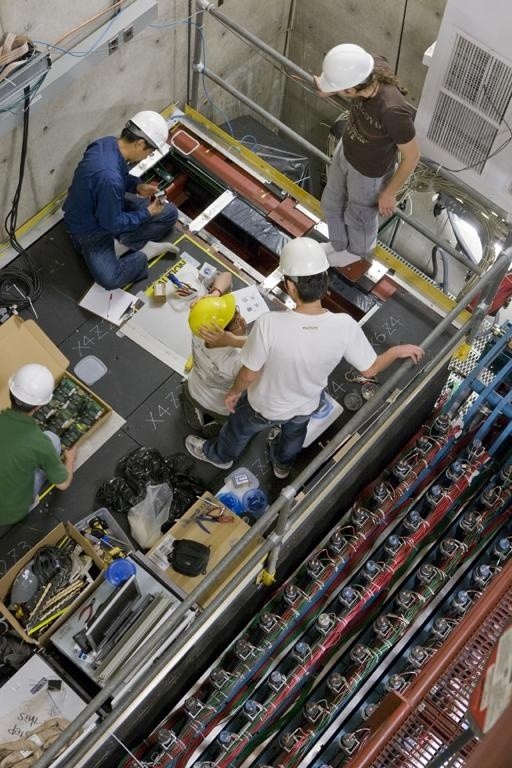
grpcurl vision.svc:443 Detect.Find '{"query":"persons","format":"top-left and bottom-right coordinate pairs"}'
top-left (0, 362), bottom-right (77, 525)
top-left (185, 237), bottom-right (421, 479)
top-left (311, 44), bottom-right (420, 269)
top-left (61, 110), bottom-right (180, 290)
top-left (177, 271), bottom-right (250, 440)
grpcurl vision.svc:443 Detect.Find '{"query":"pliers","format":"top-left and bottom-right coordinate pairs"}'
top-left (194, 517), bottom-right (217, 533)
top-left (176, 290), bottom-right (190, 297)
top-left (78, 597), bottom-right (95, 624)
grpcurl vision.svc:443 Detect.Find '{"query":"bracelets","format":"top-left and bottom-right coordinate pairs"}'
top-left (207, 286), bottom-right (222, 295)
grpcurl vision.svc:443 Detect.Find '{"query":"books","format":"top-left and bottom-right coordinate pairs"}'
top-left (72, 573), bottom-right (200, 696)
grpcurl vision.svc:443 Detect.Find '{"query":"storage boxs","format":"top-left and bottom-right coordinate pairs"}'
top-left (50, 557), bottom-right (192, 698)
top-left (0, 519), bottom-right (109, 646)
top-left (1, 314), bottom-right (112, 460)
top-left (74, 508), bottom-right (135, 566)
top-left (0, 653), bottom-right (99, 767)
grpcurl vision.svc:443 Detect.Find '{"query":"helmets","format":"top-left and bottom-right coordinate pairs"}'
top-left (130, 110), bottom-right (167, 155)
top-left (319, 42), bottom-right (375, 92)
top-left (8, 364), bottom-right (55, 406)
top-left (188, 294), bottom-right (236, 339)
top-left (276, 236), bottom-right (330, 277)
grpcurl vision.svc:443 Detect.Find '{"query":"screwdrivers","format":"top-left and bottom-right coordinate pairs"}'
top-left (175, 515), bottom-right (234, 523)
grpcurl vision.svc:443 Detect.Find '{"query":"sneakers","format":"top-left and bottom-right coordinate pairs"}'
top-left (179, 393), bottom-right (234, 470)
top-left (267, 428), bottom-right (291, 478)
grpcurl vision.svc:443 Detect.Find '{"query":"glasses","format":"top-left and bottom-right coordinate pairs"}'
top-left (228, 305), bottom-right (240, 332)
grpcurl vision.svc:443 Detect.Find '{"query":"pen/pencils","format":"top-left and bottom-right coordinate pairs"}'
top-left (106, 293), bottom-right (112, 319)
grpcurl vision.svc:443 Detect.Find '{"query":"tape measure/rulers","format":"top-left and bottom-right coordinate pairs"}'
top-left (166, 539), bottom-right (211, 577)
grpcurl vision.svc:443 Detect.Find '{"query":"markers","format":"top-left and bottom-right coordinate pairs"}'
top-left (80, 530), bottom-right (101, 545)
